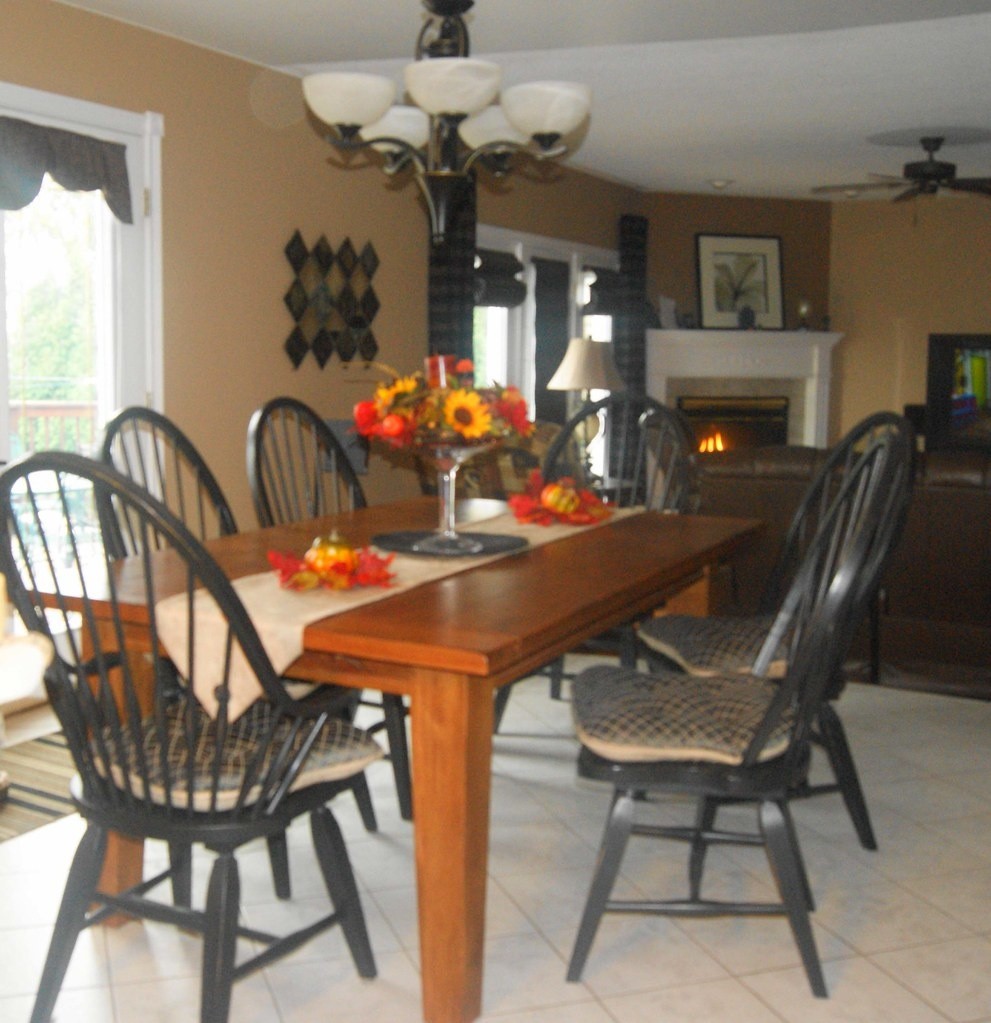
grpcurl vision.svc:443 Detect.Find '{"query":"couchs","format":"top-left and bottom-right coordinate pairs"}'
top-left (665, 445), bottom-right (991, 683)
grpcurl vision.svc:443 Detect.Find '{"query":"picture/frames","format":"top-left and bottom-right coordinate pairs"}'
top-left (695, 232), bottom-right (787, 331)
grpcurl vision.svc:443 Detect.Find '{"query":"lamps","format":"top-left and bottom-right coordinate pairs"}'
top-left (545, 335), bottom-right (626, 483)
top-left (300, 0), bottom-right (590, 250)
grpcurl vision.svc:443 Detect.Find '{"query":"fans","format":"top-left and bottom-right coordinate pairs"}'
top-left (807, 136), bottom-right (991, 205)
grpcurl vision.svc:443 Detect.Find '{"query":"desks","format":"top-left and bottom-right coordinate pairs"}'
top-left (10, 496), bottom-right (763, 1023)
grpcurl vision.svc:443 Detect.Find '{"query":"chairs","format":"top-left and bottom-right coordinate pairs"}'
top-left (246, 399), bottom-right (417, 824)
top-left (490, 394), bottom-right (703, 737)
top-left (0, 449), bottom-right (384, 1023)
top-left (93, 407), bottom-right (378, 900)
top-left (619, 406), bottom-right (919, 851)
top-left (565, 432), bottom-right (899, 1002)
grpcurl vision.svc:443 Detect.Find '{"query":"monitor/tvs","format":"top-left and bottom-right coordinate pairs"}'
top-left (924, 332), bottom-right (991, 453)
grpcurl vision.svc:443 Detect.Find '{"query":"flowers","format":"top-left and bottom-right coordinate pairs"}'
top-left (340, 354), bottom-right (539, 452)
top-left (507, 467), bottom-right (621, 528)
top-left (263, 530), bottom-right (400, 595)
